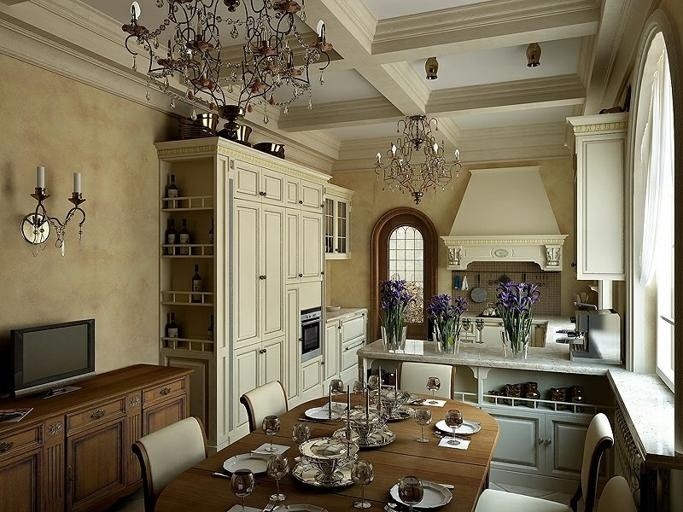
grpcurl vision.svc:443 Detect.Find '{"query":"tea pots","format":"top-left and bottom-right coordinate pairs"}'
top-left (482, 301), bottom-right (497, 317)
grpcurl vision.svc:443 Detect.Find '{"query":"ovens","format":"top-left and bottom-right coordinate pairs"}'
top-left (301, 306), bottom-right (321, 363)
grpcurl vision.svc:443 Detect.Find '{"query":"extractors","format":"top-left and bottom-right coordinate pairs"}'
top-left (435, 165), bottom-right (567, 274)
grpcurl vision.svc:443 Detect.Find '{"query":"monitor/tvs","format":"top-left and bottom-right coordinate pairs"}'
top-left (10, 319), bottom-right (95, 399)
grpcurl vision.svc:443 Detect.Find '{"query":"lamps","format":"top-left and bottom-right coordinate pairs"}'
top-left (525, 42), bottom-right (541, 68)
top-left (373, 116), bottom-right (462, 204)
top-left (423, 57), bottom-right (439, 80)
top-left (120, 0), bottom-right (332, 132)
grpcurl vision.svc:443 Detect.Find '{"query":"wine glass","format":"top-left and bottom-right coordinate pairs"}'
top-left (444, 409), bottom-right (463, 445)
top-left (329, 378), bottom-right (344, 411)
top-left (397, 475), bottom-right (425, 511)
top-left (461, 317), bottom-right (472, 342)
top-left (413, 407), bottom-right (431, 443)
top-left (475, 318), bottom-right (486, 344)
top-left (352, 459), bottom-right (375, 510)
top-left (262, 416), bottom-right (281, 452)
top-left (425, 376), bottom-right (441, 404)
top-left (265, 455), bottom-right (290, 503)
top-left (229, 468), bottom-right (254, 511)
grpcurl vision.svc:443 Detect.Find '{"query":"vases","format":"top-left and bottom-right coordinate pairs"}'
top-left (498, 323), bottom-right (531, 359)
top-left (379, 323), bottom-right (407, 351)
top-left (431, 329), bottom-right (460, 354)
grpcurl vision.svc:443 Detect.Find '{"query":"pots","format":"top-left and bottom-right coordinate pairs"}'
top-left (470, 273), bottom-right (487, 302)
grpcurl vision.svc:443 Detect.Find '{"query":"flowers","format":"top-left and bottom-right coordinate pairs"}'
top-left (375, 277), bottom-right (416, 346)
top-left (424, 292), bottom-right (469, 351)
top-left (493, 279), bottom-right (543, 356)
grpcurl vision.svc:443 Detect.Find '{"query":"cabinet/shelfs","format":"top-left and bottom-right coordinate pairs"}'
top-left (287, 176), bottom-right (324, 284)
top-left (0, 389), bottom-right (68, 512)
top-left (475, 405), bottom-right (601, 497)
top-left (298, 357), bottom-right (323, 406)
top-left (139, 356), bottom-right (195, 512)
top-left (283, 289), bottom-right (299, 412)
top-left (324, 307), bottom-right (368, 396)
top-left (157, 159), bottom-right (215, 352)
top-left (63, 371), bottom-right (139, 511)
top-left (323, 182), bottom-right (355, 262)
top-left (232, 158), bottom-right (288, 441)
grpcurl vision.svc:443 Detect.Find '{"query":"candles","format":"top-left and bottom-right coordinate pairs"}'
top-left (72, 172), bottom-right (80, 192)
top-left (36, 166), bottom-right (44, 188)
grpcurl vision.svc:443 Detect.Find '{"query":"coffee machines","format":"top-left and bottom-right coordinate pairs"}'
top-left (555, 308), bottom-right (623, 367)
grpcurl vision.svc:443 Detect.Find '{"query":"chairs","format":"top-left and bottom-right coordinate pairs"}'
top-left (131, 414), bottom-right (207, 510)
top-left (239, 379), bottom-right (287, 433)
top-left (597, 474), bottom-right (637, 510)
top-left (475, 413), bottom-right (615, 511)
top-left (399, 361), bottom-right (456, 400)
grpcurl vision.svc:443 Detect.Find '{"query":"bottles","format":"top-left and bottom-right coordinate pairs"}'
top-left (190, 262), bottom-right (202, 302)
top-left (207, 315), bottom-right (213, 350)
top-left (165, 215), bottom-right (191, 256)
top-left (207, 217), bottom-right (214, 254)
top-left (167, 172), bottom-right (179, 208)
top-left (524, 380), bottom-right (541, 408)
top-left (167, 312), bottom-right (178, 350)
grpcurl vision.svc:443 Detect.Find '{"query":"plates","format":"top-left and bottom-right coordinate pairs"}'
top-left (434, 419), bottom-right (482, 435)
top-left (223, 452), bottom-right (277, 475)
top-left (389, 480), bottom-right (454, 508)
top-left (305, 407), bottom-right (339, 420)
top-left (271, 503), bottom-right (328, 512)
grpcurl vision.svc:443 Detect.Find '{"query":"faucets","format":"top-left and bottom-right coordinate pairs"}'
top-left (573, 301), bottom-right (597, 311)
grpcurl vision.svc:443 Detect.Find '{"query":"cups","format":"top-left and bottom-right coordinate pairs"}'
top-left (486, 390), bottom-right (503, 403)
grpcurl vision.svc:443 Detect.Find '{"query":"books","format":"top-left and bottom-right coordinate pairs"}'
top-left (0, 408), bottom-right (34, 423)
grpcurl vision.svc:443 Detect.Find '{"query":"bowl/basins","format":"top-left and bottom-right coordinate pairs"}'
top-left (192, 113), bottom-right (285, 158)
